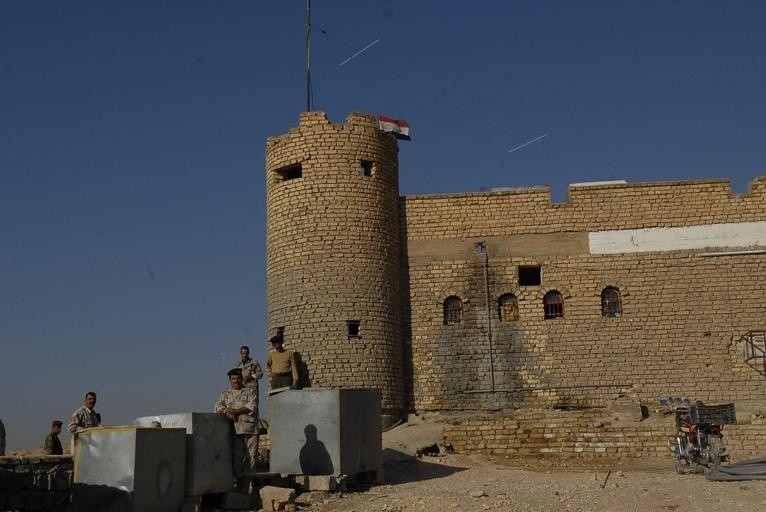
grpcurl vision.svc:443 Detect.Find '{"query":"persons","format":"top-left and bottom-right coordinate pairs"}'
top-left (0, 417), bottom-right (6, 457)
top-left (43, 420), bottom-right (64, 456)
top-left (266, 335), bottom-right (299, 390)
top-left (213, 367), bottom-right (261, 494)
top-left (64, 390), bottom-right (103, 456)
top-left (231, 344), bottom-right (263, 405)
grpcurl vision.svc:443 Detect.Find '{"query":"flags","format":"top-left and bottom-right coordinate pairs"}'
top-left (377, 112), bottom-right (412, 141)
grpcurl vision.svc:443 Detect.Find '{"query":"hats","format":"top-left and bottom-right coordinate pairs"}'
top-left (268, 334), bottom-right (284, 343)
top-left (51, 420), bottom-right (64, 427)
top-left (226, 367), bottom-right (242, 377)
